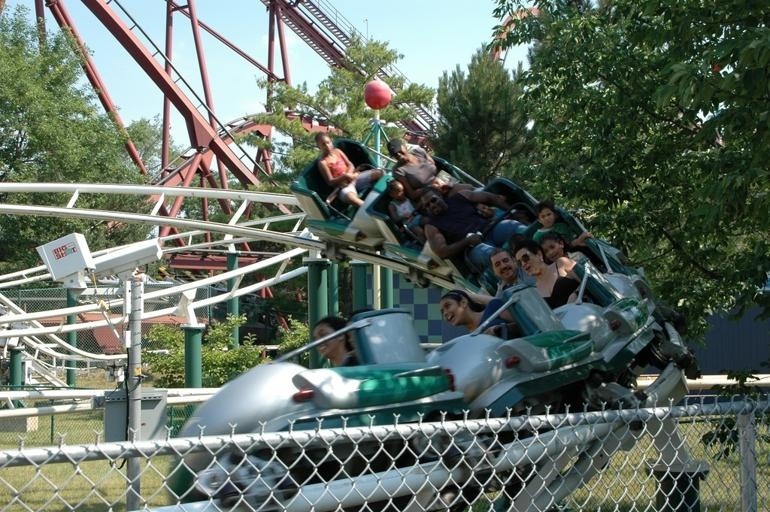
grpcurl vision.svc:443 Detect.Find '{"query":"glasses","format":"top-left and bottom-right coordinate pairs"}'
top-left (424, 195), bottom-right (438, 208)
top-left (519, 252), bottom-right (530, 269)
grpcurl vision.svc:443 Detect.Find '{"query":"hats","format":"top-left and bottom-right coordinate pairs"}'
top-left (387, 139), bottom-right (401, 157)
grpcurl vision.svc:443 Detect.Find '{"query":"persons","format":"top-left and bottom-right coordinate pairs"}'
top-left (315, 132), bottom-right (384, 206)
top-left (115, 366), bottom-right (124, 388)
top-left (313, 317), bottom-right (361, 367)
top-left (386, 139), bottom-right (608, 341)
